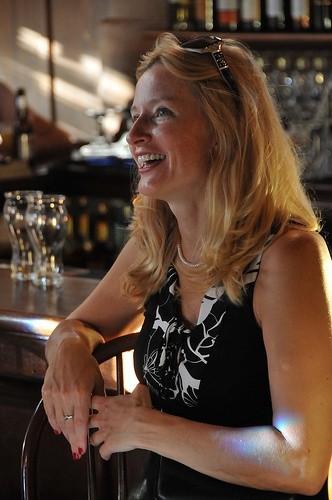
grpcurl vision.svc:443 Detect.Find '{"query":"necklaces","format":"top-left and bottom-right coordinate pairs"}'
top-left (177, 239), bottom-right (214, 267)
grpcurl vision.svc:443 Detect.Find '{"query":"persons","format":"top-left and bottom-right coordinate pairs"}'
top-left (41, 35), bottom-right (332, 500)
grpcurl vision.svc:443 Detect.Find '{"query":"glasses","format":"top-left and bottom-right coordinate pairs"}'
top-left (179, 34), bottom-right (240, 101)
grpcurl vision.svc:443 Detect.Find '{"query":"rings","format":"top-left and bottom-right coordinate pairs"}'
top-left (63, 415), bottom-right (75, 421)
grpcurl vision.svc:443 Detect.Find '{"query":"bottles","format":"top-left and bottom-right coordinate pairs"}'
top-left (254, 49), bottom-right (332, 140)
top-left (78, 195), bottom-right (116, 268)
top-left (12, 89), bottom-right (33, 160)
top-left (167, 0), bottom-right (332, 33)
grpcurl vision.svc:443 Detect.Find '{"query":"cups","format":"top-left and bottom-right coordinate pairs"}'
top-left (25, 194), bottom-right (70, 288)
top-left (3, 190), bottom-right (43, 282)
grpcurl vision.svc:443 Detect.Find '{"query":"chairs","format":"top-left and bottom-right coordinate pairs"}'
top-left (19, 333), bottom-right (156, 500)
